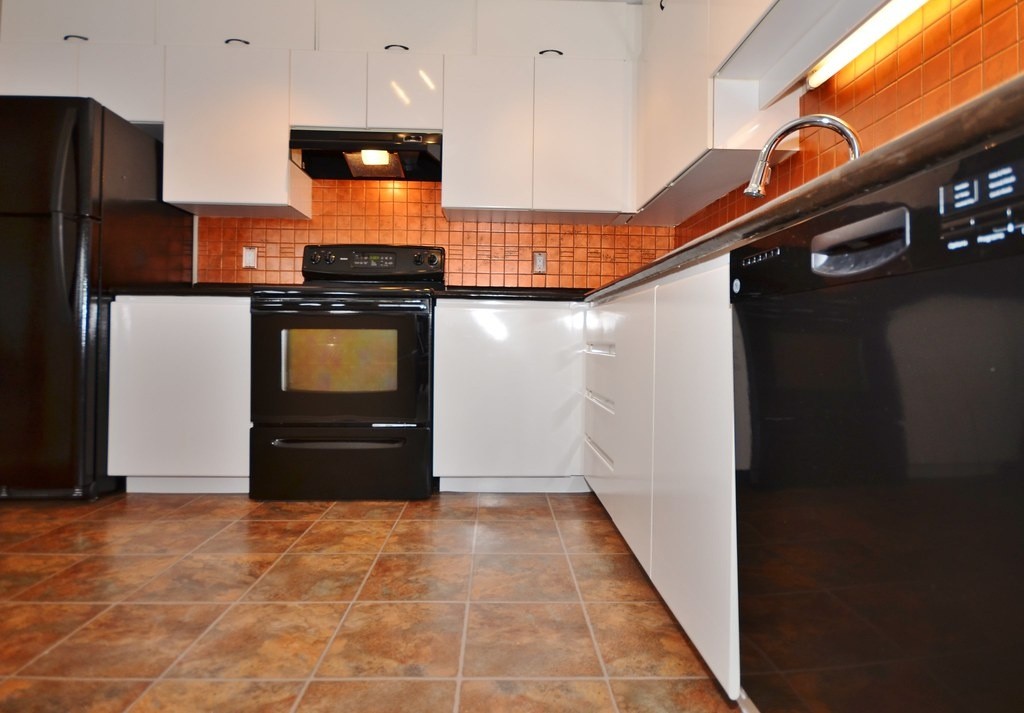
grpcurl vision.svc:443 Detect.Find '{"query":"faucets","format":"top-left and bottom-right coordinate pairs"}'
top-left (743, 113), bottom-right (863, 199)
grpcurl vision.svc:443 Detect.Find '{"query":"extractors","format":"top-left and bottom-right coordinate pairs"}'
top-left (288, 130), bottom-right (442, 182)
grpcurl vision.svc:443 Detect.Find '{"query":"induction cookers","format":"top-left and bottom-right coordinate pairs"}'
top-left (252, 244), bottom-right (444, 298)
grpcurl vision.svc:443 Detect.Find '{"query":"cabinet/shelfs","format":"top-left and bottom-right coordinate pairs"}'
top-left (634, 1), bottom-right (800, 229)
top-left (163, 46), bottom-right (312, 220)
top-left (443, 53), bottom-right (635, 225)
top-left (649, 228), bottom-right (741, 703)
top-left (0, 43), bottom-right (164, 125)
top-left (583, 261), bottom-right (656, 582)
top-left (475, 0), bottom-right (638, 55)
top-left (156, 0), bottom-right (317, 46)
top-left (108, 292), bottom-right (251, 493)
top-left (316, 0), bottom-right (476, 52)
top-left (433, 298), bottom-right (586, 493)
top-left (290, 48), bottom-right (442, 132)
top-left (1, 1), bottom-right (156, 43)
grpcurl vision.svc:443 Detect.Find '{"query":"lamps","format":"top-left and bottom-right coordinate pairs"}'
top-left (805, 0), bottom-right (928, 90)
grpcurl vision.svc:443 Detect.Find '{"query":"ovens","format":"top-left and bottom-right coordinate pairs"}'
top-left (248, 283), bottom-right (434, 500)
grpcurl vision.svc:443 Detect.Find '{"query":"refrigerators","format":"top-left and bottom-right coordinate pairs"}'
top-left (0, 95), bottom-right (163, 503)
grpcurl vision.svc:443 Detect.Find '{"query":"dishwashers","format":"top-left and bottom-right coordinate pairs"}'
top-left (729, 131), bottom-right (1024, 713)
